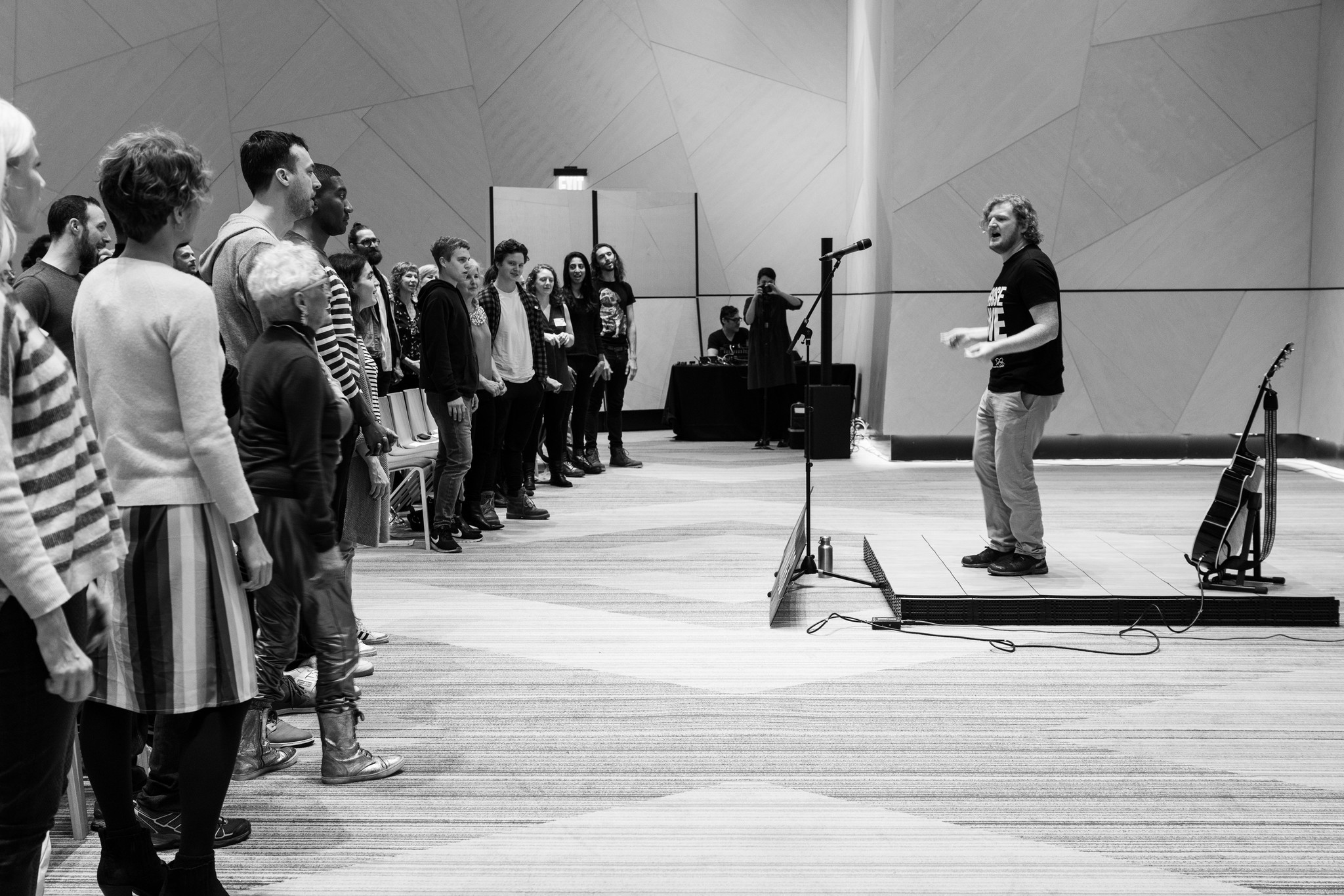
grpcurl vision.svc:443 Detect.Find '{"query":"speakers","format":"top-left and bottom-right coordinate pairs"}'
top-left (805, 384), bottom-right (852, 460)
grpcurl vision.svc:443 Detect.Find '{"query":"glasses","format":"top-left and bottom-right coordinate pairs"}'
top-left (292, 274), bottom-right (329, 294)
top-left (354, 238), bottom-right (380, 248)
top-left (724, 317), bottom-right (742, 323)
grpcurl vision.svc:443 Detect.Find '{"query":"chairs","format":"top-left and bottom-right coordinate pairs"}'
top-left (376, 387), bottom-right (440, 551)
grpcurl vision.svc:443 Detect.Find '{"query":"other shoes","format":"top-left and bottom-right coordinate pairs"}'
top-left (89, 801), bottom-right (108, 831)
top-left (777, 437), bottom-right (793, 447)
top-left (755, 437), bottom-right (770, 446)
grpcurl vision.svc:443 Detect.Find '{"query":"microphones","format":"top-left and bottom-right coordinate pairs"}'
top-left (819, 238), bottom-right (872, 261)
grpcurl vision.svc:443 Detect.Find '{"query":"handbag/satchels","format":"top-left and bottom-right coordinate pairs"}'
top-left (407, 496), bottom-right (434, 531)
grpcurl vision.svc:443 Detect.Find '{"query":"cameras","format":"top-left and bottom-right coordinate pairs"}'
top-left (759, 284), bottom-right (772, 294)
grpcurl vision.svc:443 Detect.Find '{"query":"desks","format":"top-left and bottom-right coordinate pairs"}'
top-left (662, 364), bottom-right (857, 441)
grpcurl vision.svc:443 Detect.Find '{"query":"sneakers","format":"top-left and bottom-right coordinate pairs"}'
top-left (430, 525), bottom-right (462, 552)
top-left (133, 799), bottom-right (251, 849)
top-left (451, 523), bottom-right (483, 541)
top-left (962, 546), bottom-right (1008, 568)
top-left (265, 614), bottom-right (389, 748)
top-left (987, 553), bottom-right (1048, 575)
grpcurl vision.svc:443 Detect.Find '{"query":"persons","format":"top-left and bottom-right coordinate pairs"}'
top-left (525, 264), bottom-right (575, 490)
top-left (559, 251), bottom-right (605, 477)
top-left (584, 243), bottom-right (642, 473)
top-left (707, 305), bottom-right (748, 356)
top-left (0, 92), bottom-right (563, 896)
top-left (743, 267), bottom-right (803, 448)
top-left (940, 194), bottom-right (1064, 576)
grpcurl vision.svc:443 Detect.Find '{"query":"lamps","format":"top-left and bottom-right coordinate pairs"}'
top-left (551, 165), bottom-right (588, 191)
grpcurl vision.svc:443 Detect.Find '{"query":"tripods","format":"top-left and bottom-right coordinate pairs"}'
top-left (766, 260), bottom-right (883, 592)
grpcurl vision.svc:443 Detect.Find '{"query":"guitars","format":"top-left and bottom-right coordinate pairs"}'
top-left (1189, 341), bottom-right (1296, 573)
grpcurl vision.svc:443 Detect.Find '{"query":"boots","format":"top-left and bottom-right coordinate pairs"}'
top-left (480, 491), bottom-right (500, 524)
top-left (586, 447), bottom-right (607, 471)
top-left (523, 462), bottom-right (536, 490)
top-left (161, 850), bottom-right (230, 896)
top-left (461, 501), bottom-right (504, 530)
top-left (493, 484), bottom-right (509, 507)
top-left (231, 708), bottom-right (298, 781)
top-left (571, 448), bottom-right (602, 473)
top-left (609, 446), bottom-right (644, 468)
top-left (97, 828), bottom-right (167, 896)
top-left (455, 499), bottom-right (481, 533)
top-left (548, 455), bottom-right (573, 487)
top-left (506, 486), bottom-right (550, 519)
top-left (562, 450), bottom-right (585, 477)
top-left (317, 708), bottom-right (405, 784)
